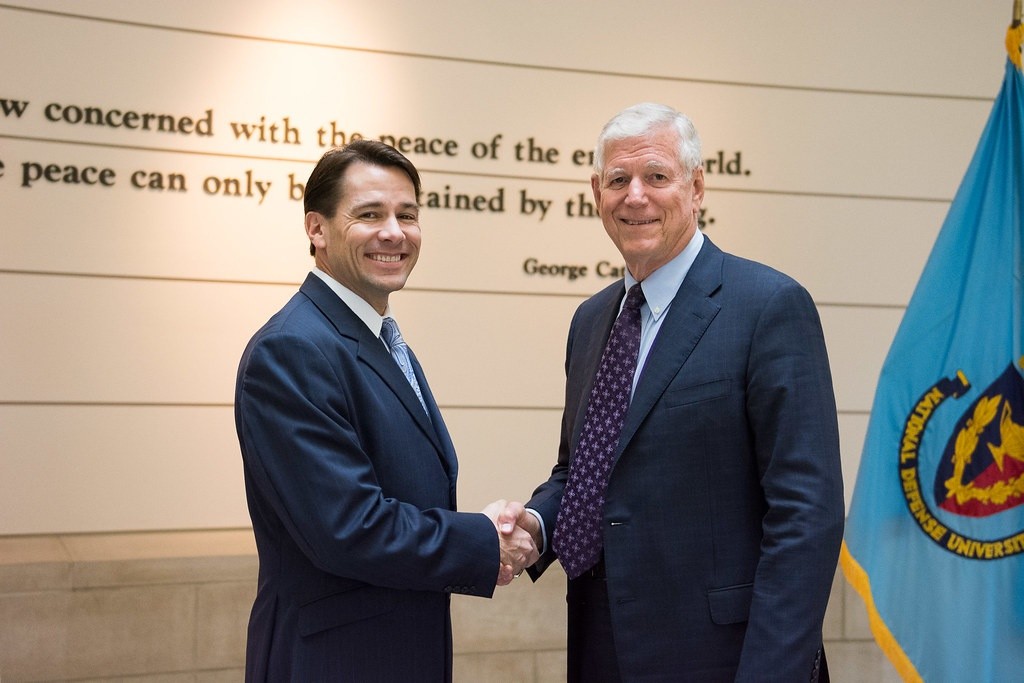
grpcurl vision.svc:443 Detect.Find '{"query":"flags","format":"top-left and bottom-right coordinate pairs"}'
top-left (838, 24), bottom-right (1024, 683)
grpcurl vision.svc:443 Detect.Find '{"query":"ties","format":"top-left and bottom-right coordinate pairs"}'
top-left (380, 317), bottom-right (432, 428)
top-left (553, 283), bottom-right (647, 582)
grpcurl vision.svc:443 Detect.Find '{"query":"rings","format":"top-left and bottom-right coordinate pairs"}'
top-left (514, 569), bottom-right (524, 578)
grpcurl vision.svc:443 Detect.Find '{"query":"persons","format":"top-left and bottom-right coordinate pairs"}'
top-left (234, 141), bottom-right (538, 683)
top-left (497, 102), bottom-right (845, 683)
